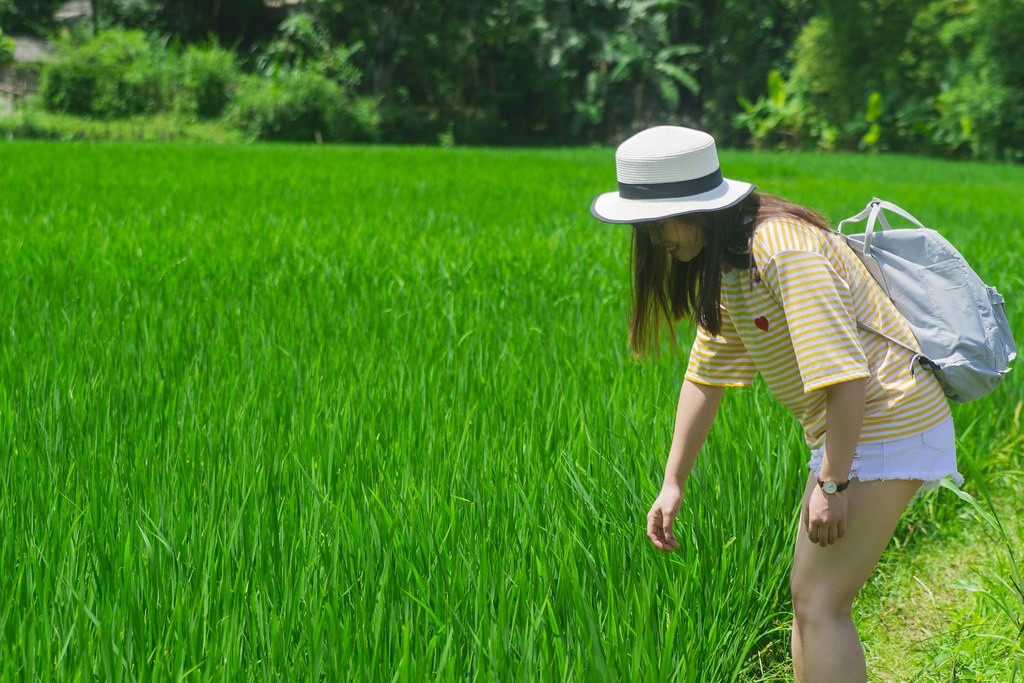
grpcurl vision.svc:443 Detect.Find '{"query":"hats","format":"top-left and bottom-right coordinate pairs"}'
top-left (588, 125), bottom-right (755, 224)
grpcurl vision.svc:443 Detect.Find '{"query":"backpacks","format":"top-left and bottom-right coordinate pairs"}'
top-left (790, 197), bottom-right (1017, 403)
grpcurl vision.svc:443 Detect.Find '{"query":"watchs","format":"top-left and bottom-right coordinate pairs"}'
top-left (816, 477), bottom-right (851, 494)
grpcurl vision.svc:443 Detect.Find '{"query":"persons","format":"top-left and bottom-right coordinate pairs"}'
top-left (591, 126), bottom-right (964, 683)
